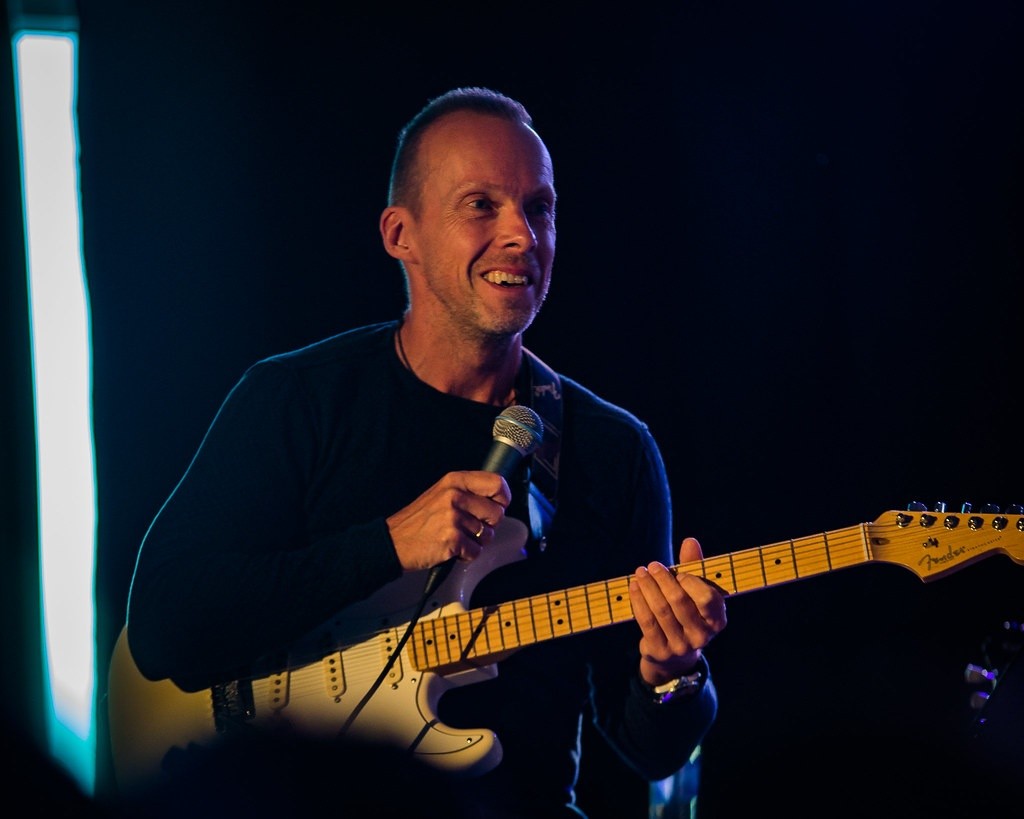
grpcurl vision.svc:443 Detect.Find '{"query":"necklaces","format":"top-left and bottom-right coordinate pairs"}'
top-left (397, 326), bottom-right (517, 407)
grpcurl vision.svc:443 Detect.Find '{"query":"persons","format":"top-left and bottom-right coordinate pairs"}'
top-left (126, 88), bottom-right (728, 819)
top-left (966, 637), bottom-right (1024, 819)
top-left (0, 713), bottom-right (1024, 819)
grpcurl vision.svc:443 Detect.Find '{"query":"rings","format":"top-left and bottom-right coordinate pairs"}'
top-left (476, 521), bottom-right (484, 538)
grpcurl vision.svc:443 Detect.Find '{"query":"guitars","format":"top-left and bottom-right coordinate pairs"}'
top-left (106, 500), bottom-right (1024, 782)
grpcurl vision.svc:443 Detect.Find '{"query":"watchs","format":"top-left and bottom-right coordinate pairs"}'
top-left (641, 656), bottom-right (704, 704)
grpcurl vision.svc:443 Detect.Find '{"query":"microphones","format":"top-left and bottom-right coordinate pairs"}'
top-left (421, 405), bottom-right (542, 596)
